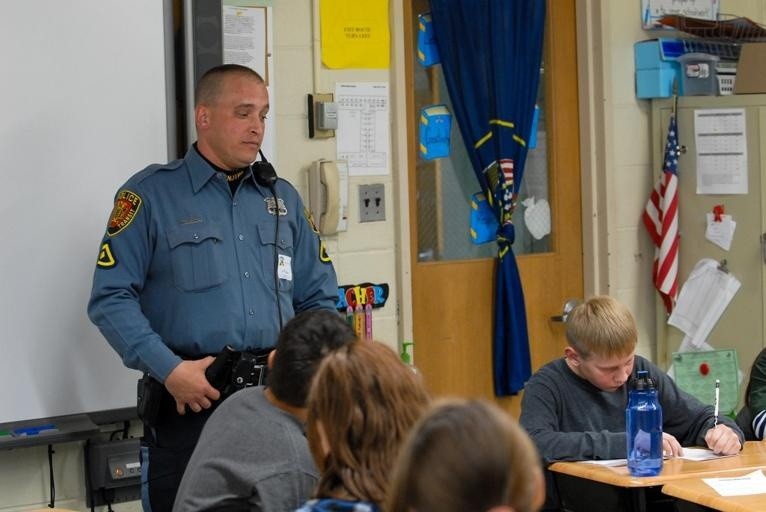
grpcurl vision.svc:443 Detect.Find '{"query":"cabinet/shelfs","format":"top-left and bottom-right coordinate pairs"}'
top-left (649, 95), bottom-right (766, 416)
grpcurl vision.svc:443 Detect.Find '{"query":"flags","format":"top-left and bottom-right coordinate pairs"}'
top-left (635, 100), bottom-right (681, 321)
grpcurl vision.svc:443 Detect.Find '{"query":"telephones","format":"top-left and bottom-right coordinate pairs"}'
top-left (307, 160), bottom-right (350, 236)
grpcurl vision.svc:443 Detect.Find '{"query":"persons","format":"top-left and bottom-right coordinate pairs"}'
top-left (518, 295), bottom-right (747, 468)
top-left (84, 63), bottom-right (342, 511)
top-left (172, 307), bottom-right (359, 512)
top-left (284, 340), bottom-right (434, 512)
top-left (382, 394), bottom-right (547, 512)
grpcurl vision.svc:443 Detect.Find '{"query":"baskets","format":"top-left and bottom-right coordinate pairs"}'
top-left (657, 11), bottom-right (762, 57)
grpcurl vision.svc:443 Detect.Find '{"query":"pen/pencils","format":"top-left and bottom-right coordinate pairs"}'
top-left (714, 380), bottom-right (721, 425)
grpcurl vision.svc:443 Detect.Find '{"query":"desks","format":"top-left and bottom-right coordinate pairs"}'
top-left (548, 439), bottom-right (764, 512)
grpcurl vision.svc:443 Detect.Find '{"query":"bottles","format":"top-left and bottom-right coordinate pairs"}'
top-left (625, 371), bottom-right (663, 477)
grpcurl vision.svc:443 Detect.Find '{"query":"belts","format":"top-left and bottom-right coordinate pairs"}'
top-left (230, 354), bottom-right (268, 390)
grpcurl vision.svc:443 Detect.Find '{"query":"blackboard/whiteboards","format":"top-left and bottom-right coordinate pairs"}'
top-left (2, 5), bottom-right (182, 432)
